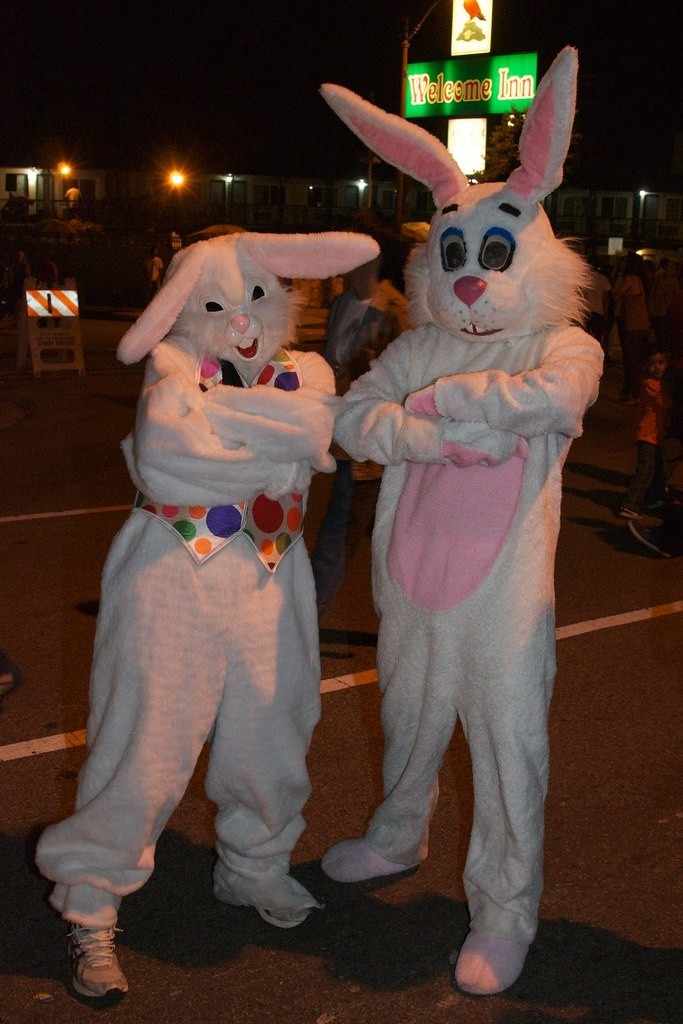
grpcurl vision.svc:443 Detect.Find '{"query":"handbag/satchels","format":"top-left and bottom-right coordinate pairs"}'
top-left (646, 326), bottom-right (656, 345)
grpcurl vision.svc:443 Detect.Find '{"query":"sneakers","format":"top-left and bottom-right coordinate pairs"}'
top-left (214, 885), bottom-right (313, 929)
top-left (628, 519), bottom-right (671, 559)
top-left (68, 923), bottom-right (129, 1002)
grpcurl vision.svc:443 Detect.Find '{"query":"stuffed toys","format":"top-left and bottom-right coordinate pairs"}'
top-left (35, 231), bottom-right (379, 1007)
top-left (321, 46), bottom-right (604, 995)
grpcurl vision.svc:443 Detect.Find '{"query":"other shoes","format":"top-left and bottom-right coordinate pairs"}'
top-left (620, 510), bottom-right (640, 519)
top-left (621, 397), bottom-right (641, 405)
top-left (647, 500), bottom-right (663, 508)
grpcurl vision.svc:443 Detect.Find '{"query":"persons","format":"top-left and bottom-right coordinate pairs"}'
top-left (143, 248), bottom-right (163, 300)
top-left (620, 348), bottom-right (679, 519)
top-left (65, 184), bottom-right (82, 208)
top-left (629, 507), bottom-right (683, 557)
top-left (583, 251), bottom-right (683, 406)
top-left (8, 249), bottom-right (32, 315)
top-left (38, 248), bottom-right (60, 328)
top-left (311, 206), bottom-right (419, 619)
